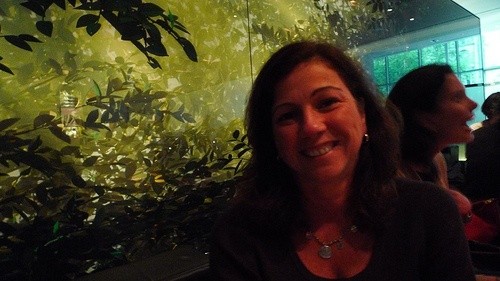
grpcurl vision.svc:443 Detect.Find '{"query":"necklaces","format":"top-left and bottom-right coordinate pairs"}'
top-left (299, 216), bottom-right (359, 259)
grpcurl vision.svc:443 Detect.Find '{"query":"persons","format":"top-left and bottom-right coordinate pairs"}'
top-left (466, 91), bottom-right (500, 197)
top-left (207, 36), bottom-right (477, 281)
top-left (384, 62), bottom-right (479, 224)
